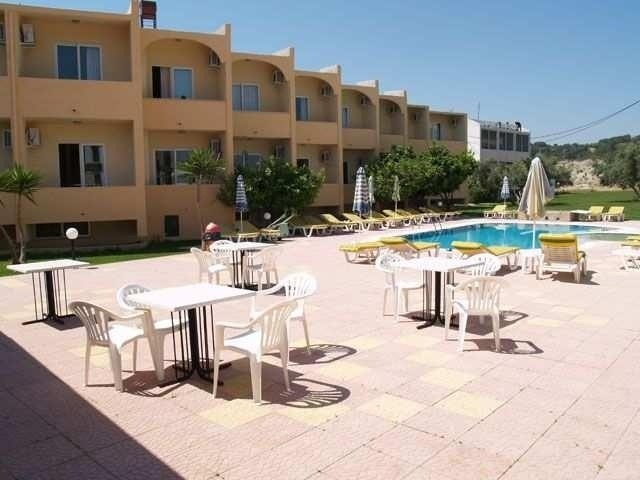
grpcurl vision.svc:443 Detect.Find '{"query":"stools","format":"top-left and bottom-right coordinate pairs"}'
top-left (622, 233), bottom-right (640, 248)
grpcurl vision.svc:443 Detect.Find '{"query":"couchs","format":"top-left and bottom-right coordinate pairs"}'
top-left (290, 216), bottom-right (329, 237)
top-left (320, 213), bottom-right (357, 234)
top-left (587, 206), bottom-right (604, 222)
top-left (217, 223), bottom-right (258, 243)
top-left (483, 205), bottom-right (507, 218)
top-left (381, 237), bottom-right (440, 258)
top-left (451, 239), bottom-right (521, 271)
top-left (361, 206), bottom-right (464, 230)
top-left (342, 213), bottom-right (375, 231)
top-left (233, 221), bottom-right (281, 241)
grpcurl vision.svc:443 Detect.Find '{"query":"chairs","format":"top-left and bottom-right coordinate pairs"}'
top-left (443, 277), bottom-right (505, 353)
top-left (535, 232), bottom-right (588, 284)
top-left (67, 301), bottom-right (166, 392)
top-left (242, 245), bottom-right (282, 292)
top-left (190, 247), bottom-right (236, 289)
top-left (210, 240), bottom-right (240, 285)
top-left (117, 284), bottom-right (195, 381)
top-left (252, 272), bottom-right (317, 357)
top-left (376, 251), bottom-right (425, 317)
top-left (210, 300), bottom-right (299, 404)
top-left (465, 253), bottom-right (501, 283)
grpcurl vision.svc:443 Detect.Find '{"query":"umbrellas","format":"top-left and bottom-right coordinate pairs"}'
top-left (518, 157), bottom-right (554, 248)
top-left (391, 175), bottom-right (401, 216)
top-left (500, 175), bottom-right (510, 211)
top-left (368, 176), bottom-right (376, 217)
top-left (235, 174), bottom-right (250, 232)
top-left (352, 166), bottom-right (371, 221)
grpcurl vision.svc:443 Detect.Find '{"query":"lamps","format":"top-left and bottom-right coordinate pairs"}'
top-left (264, 212), bottom-right (271, 221)
top-left (602, 206), bottom-right (625, 222)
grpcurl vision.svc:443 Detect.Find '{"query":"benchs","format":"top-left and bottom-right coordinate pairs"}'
top-left (339, 242), bottom-right (383, 265)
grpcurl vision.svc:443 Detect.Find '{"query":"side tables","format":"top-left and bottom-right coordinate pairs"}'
top-left (519, 251), bottom-right (540, 276)
top-left (612, 247), bottom-right (640, 270)
